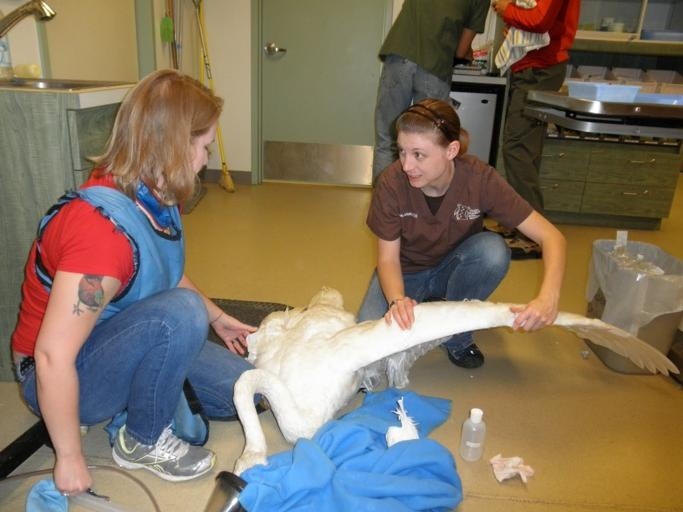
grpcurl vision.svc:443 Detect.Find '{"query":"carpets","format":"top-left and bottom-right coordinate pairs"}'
top-left (183, 299), bottom-right (294, 421)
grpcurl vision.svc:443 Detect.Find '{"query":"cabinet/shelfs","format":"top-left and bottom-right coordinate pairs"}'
top-left (0, 103), bottom-right (120, 383)
top-left (496, 138), bottom-right (683, 231)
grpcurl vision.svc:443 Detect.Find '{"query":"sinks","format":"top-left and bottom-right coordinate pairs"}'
top-left (0, 76), bottom-right (137, 91)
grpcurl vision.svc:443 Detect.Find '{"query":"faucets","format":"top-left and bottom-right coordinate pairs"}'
top-left (0, 0), bottom-right (57, 38)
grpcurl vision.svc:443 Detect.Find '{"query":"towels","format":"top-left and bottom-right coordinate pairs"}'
top-left (238, 388), bottom-right (463, 512)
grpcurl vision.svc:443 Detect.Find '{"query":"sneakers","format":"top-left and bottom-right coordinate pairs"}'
top-left (446, 339), bottom-right (484, 368)
top-left (492, 227), bottom-right (538, 251)
top-left (111, 425), bottom-right (215, 484)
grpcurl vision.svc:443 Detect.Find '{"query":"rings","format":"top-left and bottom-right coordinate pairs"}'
top-left (62, 493), bottom-right (70, 496)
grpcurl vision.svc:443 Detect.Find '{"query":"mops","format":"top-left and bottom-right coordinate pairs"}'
top-left (168, 0), bottom-right (208, 214)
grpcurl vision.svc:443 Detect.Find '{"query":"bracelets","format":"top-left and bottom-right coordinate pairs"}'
top-left (386, 297), bottom-right (404, 308)
top-left (214, 301), bottom-right (224, 325)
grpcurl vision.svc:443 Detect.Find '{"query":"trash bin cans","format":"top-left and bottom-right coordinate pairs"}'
top-left (583, 239), bottom-right (683, 375)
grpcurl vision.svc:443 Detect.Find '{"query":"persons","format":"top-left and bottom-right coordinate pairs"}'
top-left (371, 0), bottom-right (491, 195)
top-left (481, 0), bottom-right (581, 260)
top-left (341, 98), bottom-right (567, 392)
top-left (10, 70), bottom-right (260, 496)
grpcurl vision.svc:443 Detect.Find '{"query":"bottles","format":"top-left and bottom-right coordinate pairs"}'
top-left (459, 408), bottom-right (486, 463)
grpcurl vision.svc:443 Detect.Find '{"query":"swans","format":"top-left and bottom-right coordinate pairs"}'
top-left (231, 283), bottom-right (681, 478)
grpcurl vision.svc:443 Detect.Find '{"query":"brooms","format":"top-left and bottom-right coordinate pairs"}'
top-left (196, 4), bottom-right (237, 193)
